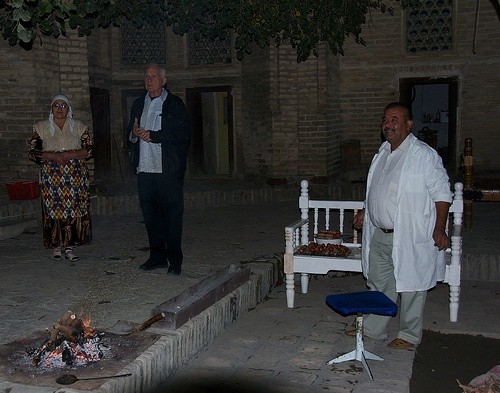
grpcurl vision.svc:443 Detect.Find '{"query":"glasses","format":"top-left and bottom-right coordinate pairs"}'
top-left (54, 104), bottom-right (68, 109)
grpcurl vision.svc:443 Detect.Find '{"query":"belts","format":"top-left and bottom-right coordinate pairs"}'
top-left (380, 228), bottom-right (393, 233)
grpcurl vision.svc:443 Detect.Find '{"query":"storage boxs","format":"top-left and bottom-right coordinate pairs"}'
top-left (6, 179), bottom-right (40, 201)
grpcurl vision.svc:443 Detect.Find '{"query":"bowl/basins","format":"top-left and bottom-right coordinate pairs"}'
top-left (316, 238), bottom-right (343, 245)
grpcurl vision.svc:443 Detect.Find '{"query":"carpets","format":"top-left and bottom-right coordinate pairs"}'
top-left (409, 329), bottom-right (500, 393)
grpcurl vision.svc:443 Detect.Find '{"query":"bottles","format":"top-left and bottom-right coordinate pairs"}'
top-left (422, 111), bottom-right (440, 123)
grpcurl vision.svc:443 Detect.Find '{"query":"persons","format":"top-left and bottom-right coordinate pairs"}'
top-left (30, 95), bottom-right (93, 262)
top-left (346, 102), bottom-right (454, 350)
top-left (125, 63), bottom-right (189, 276)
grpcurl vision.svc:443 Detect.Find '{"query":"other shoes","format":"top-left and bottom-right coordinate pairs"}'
top-left (388, 338), bottom-right (414, 349)
top-left (167, 263), bottom-right (181, 277)
top-left (140, 257), bottom-right (168, 271)
top-left (345, 329), bottom-right (356, 336)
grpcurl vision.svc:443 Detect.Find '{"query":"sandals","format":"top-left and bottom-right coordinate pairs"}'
top-left (65, 251), bottom-right (80, 261)
top-left (52, 249), bottom-right (62, 260)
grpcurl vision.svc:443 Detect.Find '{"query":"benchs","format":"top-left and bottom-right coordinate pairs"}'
top-left (284, 180), bottom-right (463, 322)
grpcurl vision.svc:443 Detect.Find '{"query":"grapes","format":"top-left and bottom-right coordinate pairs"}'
top-left (299, 242), bottom-right (334, 253)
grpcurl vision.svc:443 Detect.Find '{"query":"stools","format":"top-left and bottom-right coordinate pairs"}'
top-left (326, 291), bottom-right (398, 381)
